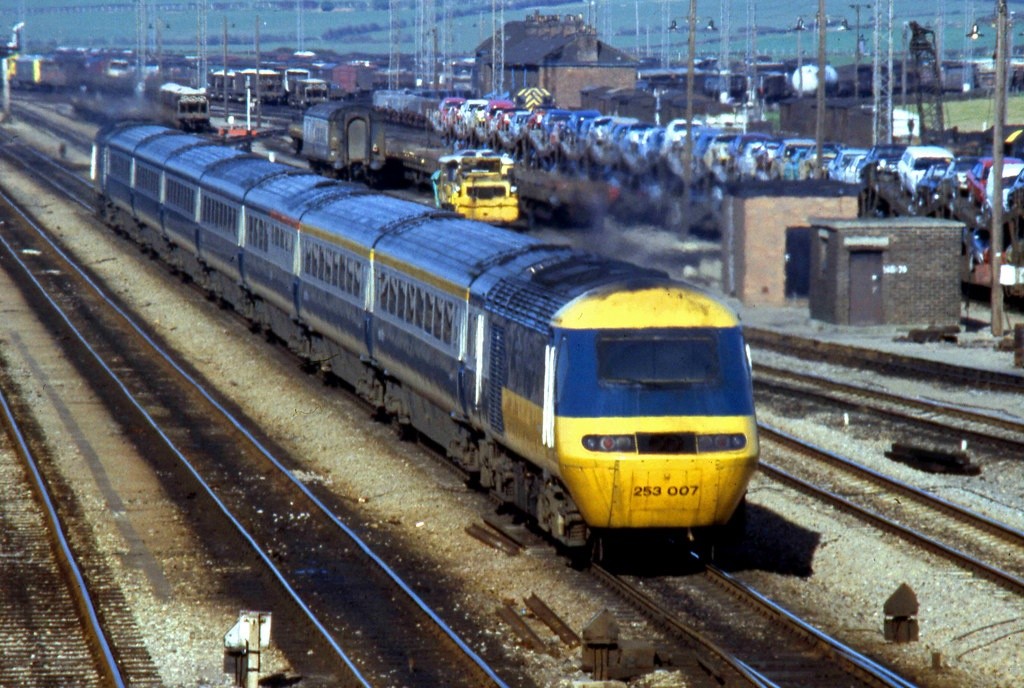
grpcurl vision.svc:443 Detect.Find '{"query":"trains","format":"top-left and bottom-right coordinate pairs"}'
top-left (85, 118), bottom-right (763, 567)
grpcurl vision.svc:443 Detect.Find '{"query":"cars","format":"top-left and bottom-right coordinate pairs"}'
top-left (158, 46), bottom-right (1024, 309)
